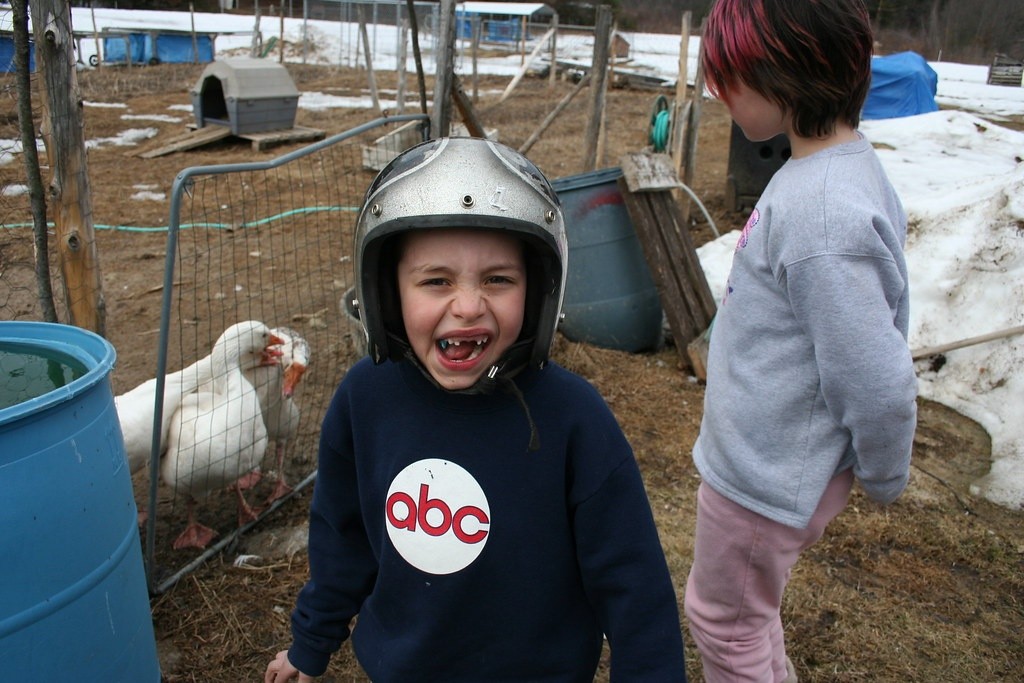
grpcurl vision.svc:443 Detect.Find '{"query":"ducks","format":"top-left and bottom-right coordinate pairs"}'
top-left (110, 320), bottom-right (312, 551)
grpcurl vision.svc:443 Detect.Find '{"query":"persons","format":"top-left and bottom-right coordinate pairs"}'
top-left (262, 136), bottom-right (689, 683)
top-left (677, 1), bottom-right (920, 683)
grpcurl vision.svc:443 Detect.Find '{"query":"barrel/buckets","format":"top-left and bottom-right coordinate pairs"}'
top-left (0, 321), bottom-right (161, 683)
top-left (549, 166), bottom-right (663, 356)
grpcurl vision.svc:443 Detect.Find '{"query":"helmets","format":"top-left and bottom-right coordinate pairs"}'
top-left (353, 137), bottom-right (569, 366)
